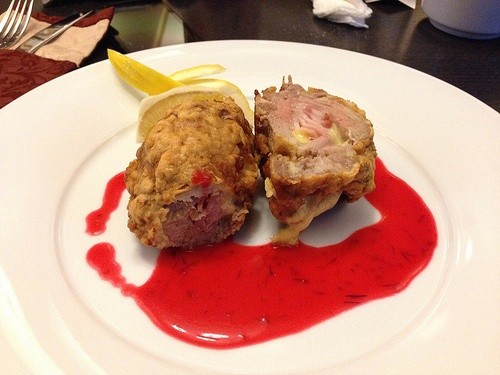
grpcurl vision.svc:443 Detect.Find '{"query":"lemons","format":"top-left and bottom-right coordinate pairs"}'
top-left (107, 49), bottom-right (254, 142)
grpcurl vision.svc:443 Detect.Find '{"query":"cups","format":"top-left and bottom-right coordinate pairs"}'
top-left (420, 0), bottom-right (500, 39)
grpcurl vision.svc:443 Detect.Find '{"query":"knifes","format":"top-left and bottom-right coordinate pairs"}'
top-left (15, 10), bottom-right (93, 53)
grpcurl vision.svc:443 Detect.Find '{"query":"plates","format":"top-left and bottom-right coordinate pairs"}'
top-left (0, 40), bottom-right (500, 375)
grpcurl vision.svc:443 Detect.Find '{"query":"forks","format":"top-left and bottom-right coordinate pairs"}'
top-left (1, 0), bottom-right (34, 48)
